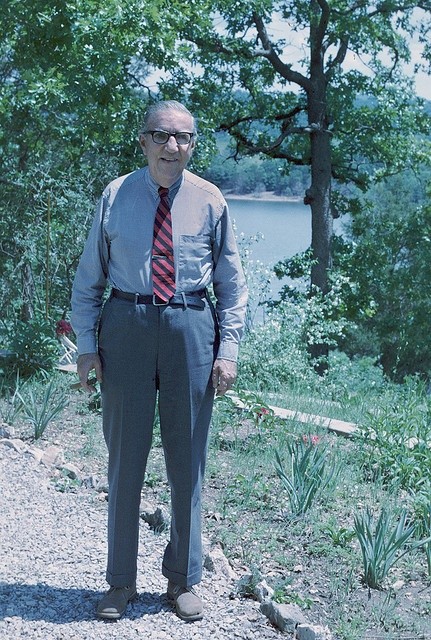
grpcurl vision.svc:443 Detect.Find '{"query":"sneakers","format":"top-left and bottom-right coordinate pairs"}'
top-left (167, 581), bottom-right (203, 621)
top-left (97, 581), bottom-right (137, 618)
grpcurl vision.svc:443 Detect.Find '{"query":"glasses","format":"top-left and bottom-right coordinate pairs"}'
top-left (141, 131), bottom-right (193, 144)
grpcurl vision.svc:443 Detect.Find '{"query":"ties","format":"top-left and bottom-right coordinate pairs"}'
top-left (152, 186), bottom-right (176, 303)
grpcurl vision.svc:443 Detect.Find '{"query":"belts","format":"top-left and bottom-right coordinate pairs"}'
top-left (112, 288), bottom-right (206, 310)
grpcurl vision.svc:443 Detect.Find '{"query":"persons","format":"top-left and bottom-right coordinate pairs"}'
top-left (70, 100), bottom-right (248, 621)
